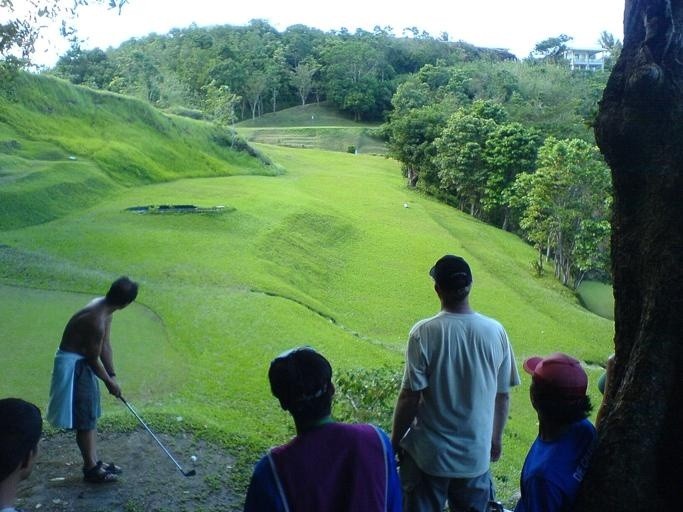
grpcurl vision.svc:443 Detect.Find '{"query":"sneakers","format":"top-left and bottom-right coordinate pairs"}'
top-left (96, 460), bottom-right (122, 474)
top-left (84, 465), bottom-right (119, 482)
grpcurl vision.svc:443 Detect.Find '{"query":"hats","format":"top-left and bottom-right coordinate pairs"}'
top-left (522, 352), bottom-right (588, 397)
top-left (268, 345), bottom-right (333, 405)
top-left (429, 254), bottom-right (473, 291)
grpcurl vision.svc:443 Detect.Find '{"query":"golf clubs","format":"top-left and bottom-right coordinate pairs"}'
top-left (119, 396), bottom-right (196, 476)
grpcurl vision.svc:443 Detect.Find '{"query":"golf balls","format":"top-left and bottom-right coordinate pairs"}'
top-left (190, 455), bottom-right (197, 462)
top-left (404, 204), bottom-right (409, 208)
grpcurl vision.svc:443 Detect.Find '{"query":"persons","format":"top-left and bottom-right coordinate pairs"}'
top-left (389, 253), bottom-right (520, 512)
top-left (514, 351), bottom-right (596, 510)
top-left (243, 346), bottom-right (404, 512)
top-left (0, 397), bottom-right (42, 512)
top-left (44, 274), bottom-right (139, 483)
top-left (594, 353), bottom-right (615, 426)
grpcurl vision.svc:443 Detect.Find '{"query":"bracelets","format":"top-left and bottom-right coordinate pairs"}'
top-left (109, 373), bottom-right (115, 377)
